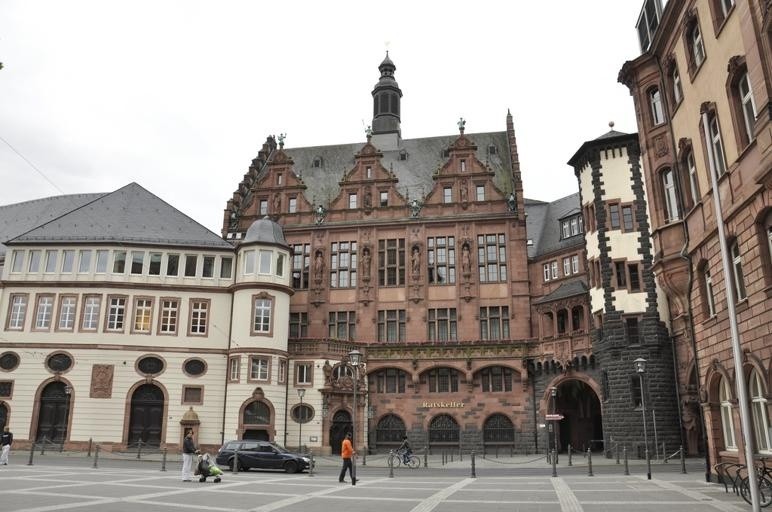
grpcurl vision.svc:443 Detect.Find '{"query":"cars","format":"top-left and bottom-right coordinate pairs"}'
top-left (215, 441), bottom-right (315, 473)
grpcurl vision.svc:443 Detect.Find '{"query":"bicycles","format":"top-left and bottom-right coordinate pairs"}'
top-left (740, 457), bottom-right (772, 508)
top-left (388, 448), bottom-right (420, 470)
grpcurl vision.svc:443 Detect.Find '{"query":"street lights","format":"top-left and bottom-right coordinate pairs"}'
top-left (296, 388), bottom-right (306, 453)
top-left (633, 355), bottom-right (651, 480)
top-left (549, 385), bottom-right (559, 465)
top-left (59, 385), bottom-right (73, 453)
top-left (349, 350), bottom-right (362, 486)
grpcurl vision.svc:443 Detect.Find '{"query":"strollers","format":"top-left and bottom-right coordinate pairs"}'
top-left (194, 451), bottom-right (221, 483)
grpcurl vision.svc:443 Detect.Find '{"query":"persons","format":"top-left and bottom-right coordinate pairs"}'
top-left (182, 430), bottom-right (200, 481)
top-left (339, 432), bottom-right (359, 483)
top-left (396, 436), bottom-right (412, 465)
top-left (0, 426), bottom-right (13, 465)
